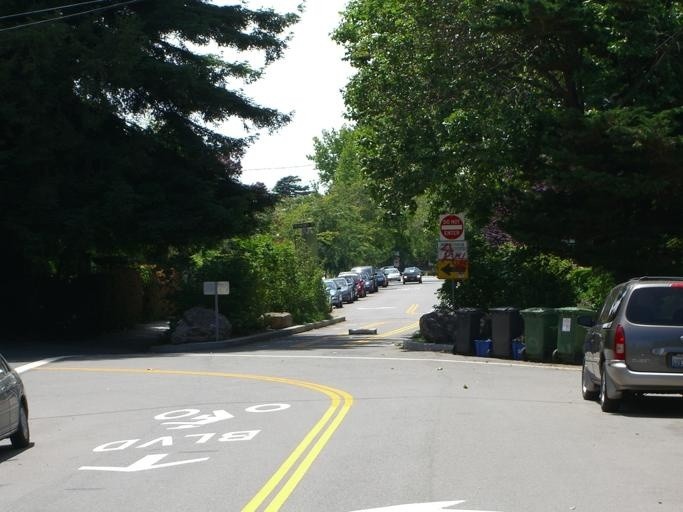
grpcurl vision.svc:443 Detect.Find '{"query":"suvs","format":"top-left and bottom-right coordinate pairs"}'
top-left (582, 275), bottom-right (683, 412)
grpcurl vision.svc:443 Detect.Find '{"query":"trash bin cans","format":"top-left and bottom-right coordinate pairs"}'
top-left (489, 307), bottom-right (521, 359)
top-left (454, 307), bottom-right (481, 356)
top-left (552, 307), bottom-right (598, 365)
top-left (518, 306), bottom-right (558, 362)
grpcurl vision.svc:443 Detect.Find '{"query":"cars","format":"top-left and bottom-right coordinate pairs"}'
top-left (0, 351), bottom-right (30, 449)
top-left (400, 267), bottom-right (421, 284)
top-left (321, 266), bottom-right (401, 308)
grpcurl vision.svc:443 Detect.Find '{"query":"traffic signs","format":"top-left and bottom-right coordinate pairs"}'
top-left (437, 241), bottom-right (469, 280)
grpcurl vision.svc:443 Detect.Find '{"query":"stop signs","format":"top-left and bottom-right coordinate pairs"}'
top-left (439, 213), bottom-right (464, 241)
